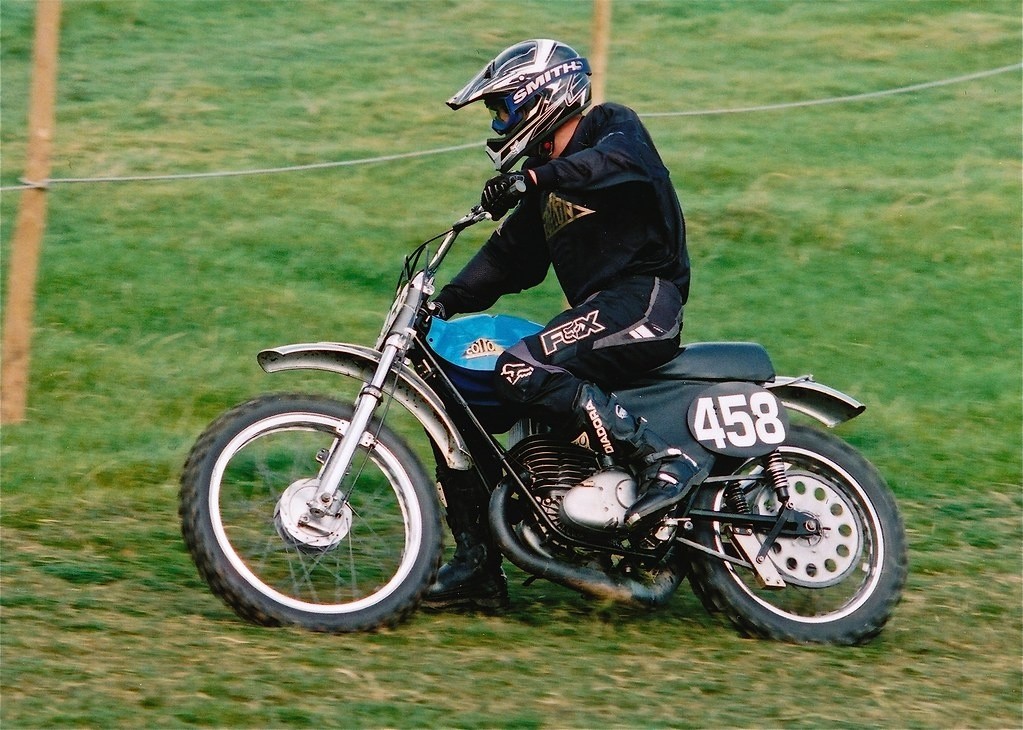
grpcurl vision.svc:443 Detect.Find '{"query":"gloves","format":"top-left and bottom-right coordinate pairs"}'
top-left (480, 170), bottom-right (525, 223)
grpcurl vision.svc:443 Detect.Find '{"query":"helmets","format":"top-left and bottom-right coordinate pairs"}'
top-left (445, 38), bottom-right (596, 173)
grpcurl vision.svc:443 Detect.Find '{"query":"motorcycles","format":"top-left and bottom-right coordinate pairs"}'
top-left (172, 180), bottom-right (910, 649)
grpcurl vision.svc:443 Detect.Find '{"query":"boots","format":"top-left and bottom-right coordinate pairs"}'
top-left (568, 378), bottom-right (715, 541)
top-left (419, 459), bottom-right (513, 612)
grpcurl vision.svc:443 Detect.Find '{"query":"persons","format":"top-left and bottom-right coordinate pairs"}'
top-left (410, 37), bottom-right (716, 602)
